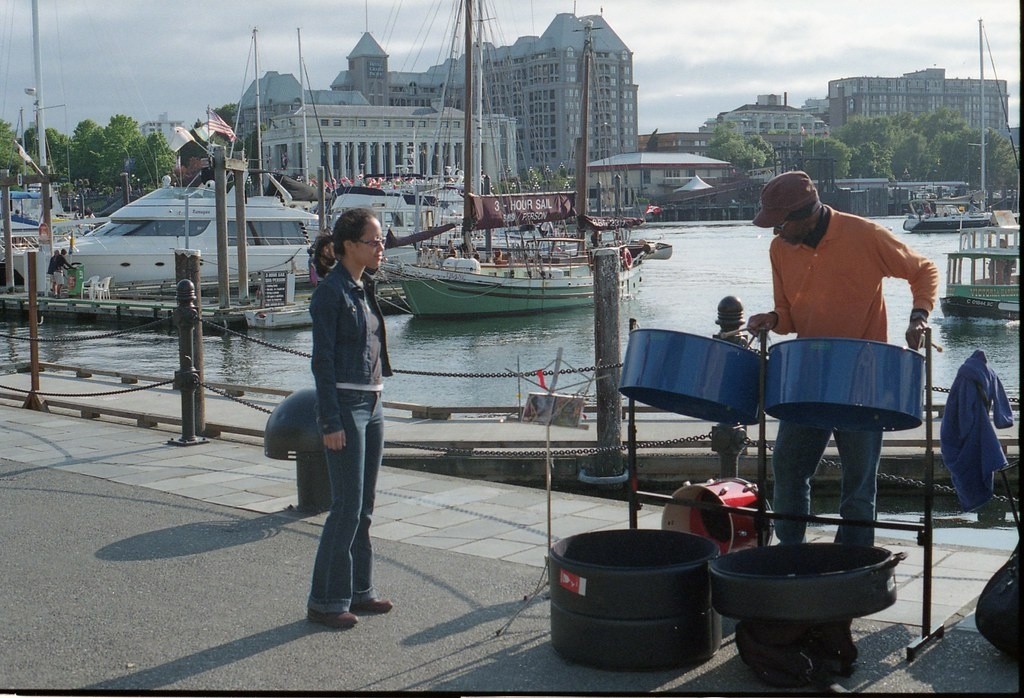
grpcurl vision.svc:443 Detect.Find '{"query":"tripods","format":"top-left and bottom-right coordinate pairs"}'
top-left (496, 347), bottom-right (601, 637)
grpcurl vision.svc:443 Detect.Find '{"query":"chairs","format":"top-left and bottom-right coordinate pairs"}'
top-left (81, 276), bottom-right (112, 301)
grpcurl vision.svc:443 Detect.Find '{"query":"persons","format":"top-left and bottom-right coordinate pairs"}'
top-left (48, 249), bottom-right (76, 297)
top-left (969, 194), bottom-right (975, 210)
top-left (305, 208), bottom-right (392, 628)
top-left (746, 171), bottom-right (938, 547)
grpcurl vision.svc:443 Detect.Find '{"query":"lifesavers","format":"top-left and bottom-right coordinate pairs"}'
top-left (39, 223), bottom-right (49, 236)
top-left (622, 248), bottom-right (633, 268)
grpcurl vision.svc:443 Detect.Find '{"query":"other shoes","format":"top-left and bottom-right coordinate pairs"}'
top-left (351, 599), bottom-right (394, 615)
top-left (307, 609), bottom-right (358, 628)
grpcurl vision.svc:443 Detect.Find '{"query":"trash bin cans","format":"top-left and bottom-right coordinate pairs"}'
top-left (67, 262), bottom-right (84, 298)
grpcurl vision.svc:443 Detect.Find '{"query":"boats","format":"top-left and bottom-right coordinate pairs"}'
top-left (939, 211), bottom-right (1021, 321)
top-left (0, 2), bottom-right (551, 299)
top-left (643, 242), bottom-right (672, 259)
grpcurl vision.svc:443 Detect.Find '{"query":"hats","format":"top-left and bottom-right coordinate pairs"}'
top-left (752, 170), bottom-right (819, 229)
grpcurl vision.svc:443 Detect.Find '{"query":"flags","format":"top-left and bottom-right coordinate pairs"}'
top-left (168, 126), bottom-right (195, 152)
top-left (195, 124), bottom-right (215, 141)
top-left (646, 205), bottom-right (661, 214)
top-left (208, 109), bottom-right (237, 142)
top-left (18, 145), bottom-right (32, 162)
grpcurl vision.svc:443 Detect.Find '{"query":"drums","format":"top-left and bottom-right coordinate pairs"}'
top-left (616, 326), bottom-right (770, 426)
top-left (762, 336), bottom-right (929, 433)
top-left (660, 475), bottom-right (774, 556)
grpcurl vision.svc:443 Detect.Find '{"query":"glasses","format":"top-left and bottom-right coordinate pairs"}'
top-left (352, 238), bottom-right (385, 248)
top-left (774, 219), bottom-right (788, 231)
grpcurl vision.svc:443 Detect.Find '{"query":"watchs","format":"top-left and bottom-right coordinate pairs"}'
top-left (909, 312), bottom-right (928, 322)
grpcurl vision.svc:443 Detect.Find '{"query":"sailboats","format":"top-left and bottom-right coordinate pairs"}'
top-left (374, 1), bottom-right (658, 320)
top-left (902, 18), bottom-right (1020, 233)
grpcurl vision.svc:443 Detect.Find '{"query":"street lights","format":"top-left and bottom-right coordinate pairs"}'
top-left (111, 143), bottom-right (131, 195)
